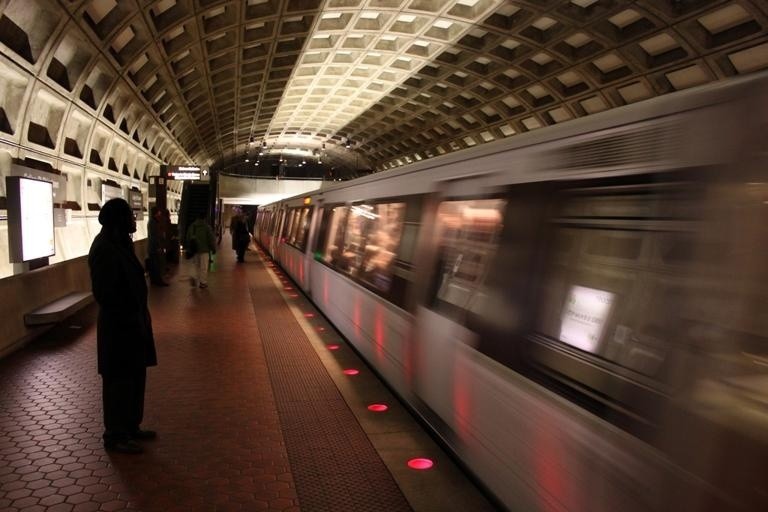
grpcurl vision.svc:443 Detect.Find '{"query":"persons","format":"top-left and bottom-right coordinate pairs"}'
top-left (147, 200), bottom-right (254, 288)
top-left (88, 198), bottom-right (156, 454)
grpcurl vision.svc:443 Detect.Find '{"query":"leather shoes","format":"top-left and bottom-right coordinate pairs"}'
top-left (102, 430), bottom-right (155, 454)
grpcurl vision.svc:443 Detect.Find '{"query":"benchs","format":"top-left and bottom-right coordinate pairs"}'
top-left (24, 291), bottom-right (98, 348)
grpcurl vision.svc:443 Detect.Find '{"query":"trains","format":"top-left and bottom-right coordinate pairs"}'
top-left (251, 70), bottom-right (767, 511)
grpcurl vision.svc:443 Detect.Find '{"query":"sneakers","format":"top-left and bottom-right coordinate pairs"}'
top-left (199, 283), bottom-right (208, 288)
